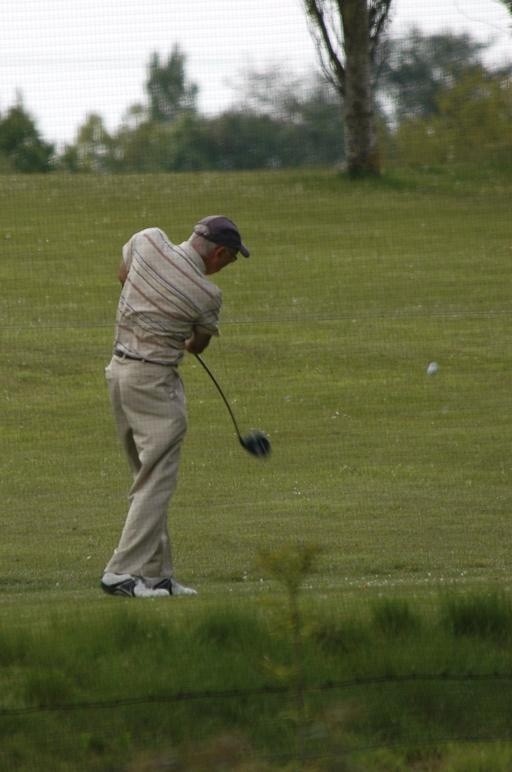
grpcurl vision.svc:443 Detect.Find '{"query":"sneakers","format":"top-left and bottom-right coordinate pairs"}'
top-left (134, 576), bottom-right (196, 596)
top-left (101, 573), bottom-right (136, 598)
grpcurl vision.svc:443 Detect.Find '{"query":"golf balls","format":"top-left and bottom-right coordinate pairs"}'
top-left (428, 362), bottom-right (440, 375)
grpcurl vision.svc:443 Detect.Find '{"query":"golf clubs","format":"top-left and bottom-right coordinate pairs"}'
top-left (194, 353), bottom-right (271, 455)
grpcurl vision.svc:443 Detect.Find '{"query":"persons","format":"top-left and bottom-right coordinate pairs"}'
top-left (99, 214), bottom-right (250, 599)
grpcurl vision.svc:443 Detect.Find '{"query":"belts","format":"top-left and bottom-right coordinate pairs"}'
top-left (114, 350), bottom-right (177, 367)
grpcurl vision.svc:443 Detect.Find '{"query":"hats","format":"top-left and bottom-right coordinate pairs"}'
top-left (194, 215), bottom-right (249, 257)
